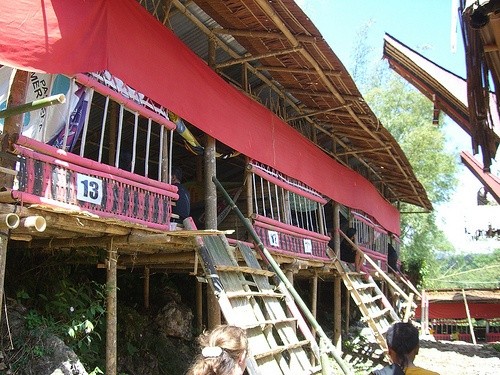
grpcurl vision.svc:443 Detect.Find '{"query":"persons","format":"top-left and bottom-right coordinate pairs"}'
top-left (183, 324), bottom-right (248, 375)
top-left (340, 226), bottom-right (365, 273)
top-left (170, 163), bottom-right (190, 225)
top-left (388, 242), bottom-right (398, 273)
top-left (368, 322), bottom-right (441, 375)
